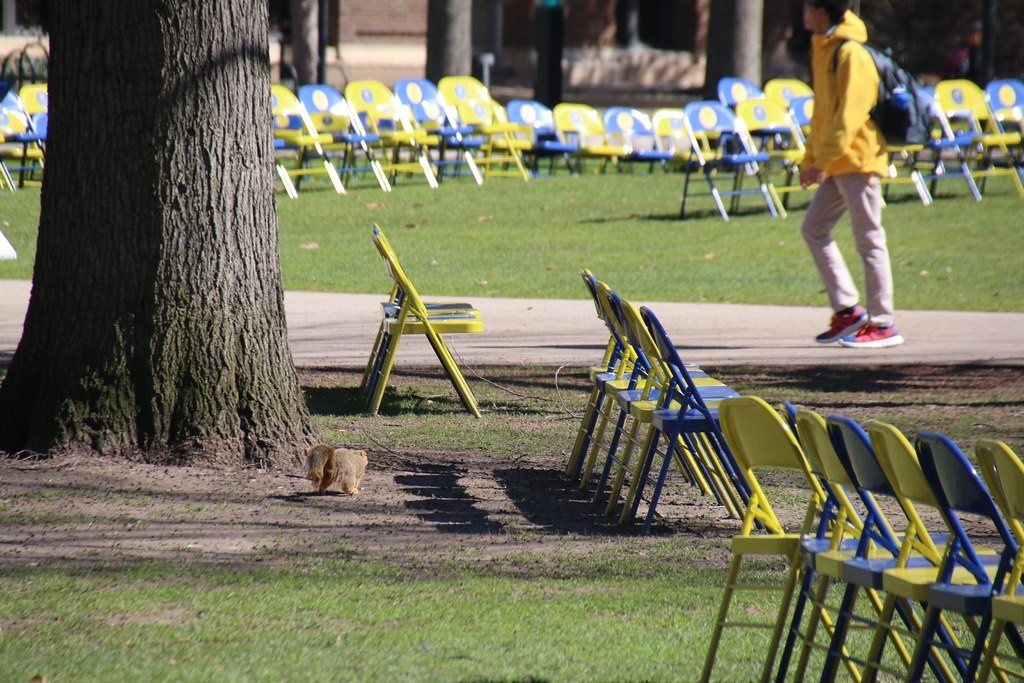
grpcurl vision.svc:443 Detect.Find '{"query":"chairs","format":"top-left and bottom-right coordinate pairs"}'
top-left (562, 265), bottom-right (1024, 683)
top-left (358, 222), bottom-right (485, 420)
top-left (0, 65), bottom-right (1024, 226)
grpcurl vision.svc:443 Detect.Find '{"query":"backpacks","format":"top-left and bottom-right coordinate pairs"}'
top-left (830, 38), bottom-right (937, 146)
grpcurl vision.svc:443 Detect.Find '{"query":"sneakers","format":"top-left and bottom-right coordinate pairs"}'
top-left (815, 303), bottom-right (869, 344)
top-left (838, 323), bottom-right (904, 349)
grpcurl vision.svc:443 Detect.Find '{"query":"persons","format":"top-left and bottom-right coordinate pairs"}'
top-left (798, 0), bottom-right (902, 349)
